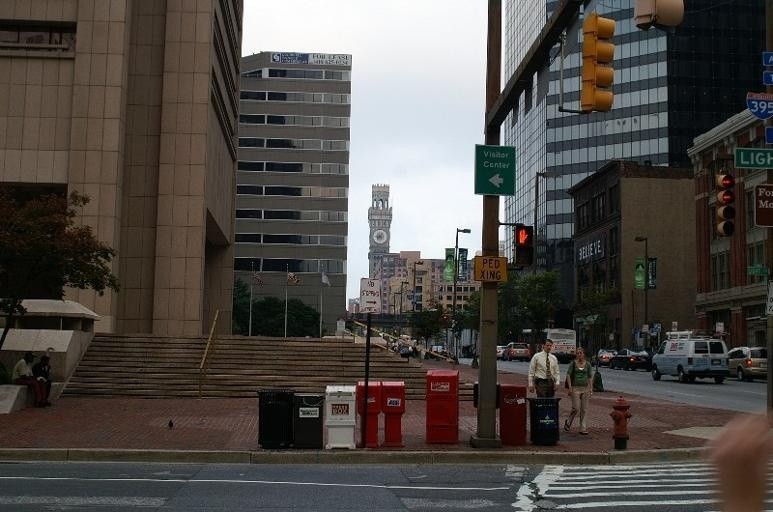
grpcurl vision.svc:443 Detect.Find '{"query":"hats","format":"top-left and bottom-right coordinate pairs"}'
top-left (22, 352), bottom-right (38, 359)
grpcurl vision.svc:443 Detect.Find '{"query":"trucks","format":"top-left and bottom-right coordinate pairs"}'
top-left (429, 345), bottom-right (442, 352)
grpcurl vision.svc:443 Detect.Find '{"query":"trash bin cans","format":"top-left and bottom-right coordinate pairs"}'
top-left (526, 397), bottom-right (562, 445)
top-left (257, 388), bottom-right (295, 449)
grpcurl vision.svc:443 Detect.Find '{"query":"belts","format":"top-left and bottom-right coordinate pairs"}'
top-left (536, 377), bottom-right (554, 383)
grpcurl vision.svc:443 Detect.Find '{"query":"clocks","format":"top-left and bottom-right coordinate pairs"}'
top-left (372, 229), bottom-right (388, 244)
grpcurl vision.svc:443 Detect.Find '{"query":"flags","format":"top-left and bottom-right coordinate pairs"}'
top-left (287, 271), bottom-right (303, 286)
top-left (253, 272), bottom-right (264, 289)
top-left (320, 270), bottom-right (332, 287)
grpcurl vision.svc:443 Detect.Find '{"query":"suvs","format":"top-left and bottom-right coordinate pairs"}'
top-left (501, 340), bottom-right (529, 360)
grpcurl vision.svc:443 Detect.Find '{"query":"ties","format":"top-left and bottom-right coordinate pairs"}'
top-left (545, 351), bottom-right (552, 380)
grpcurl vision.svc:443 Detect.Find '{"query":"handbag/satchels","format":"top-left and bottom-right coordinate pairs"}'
top-left (564, 360), bottom-right (576, 389)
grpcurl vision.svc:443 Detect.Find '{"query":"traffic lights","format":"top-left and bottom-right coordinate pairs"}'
top-left (580, 14), bottom-right (620, 113)
top-left (633, 0), bottom-right (685, 32)
top-left (512, 223), bottom-right (535, 266)
top-left (713, 173), bottom-right (734, 235)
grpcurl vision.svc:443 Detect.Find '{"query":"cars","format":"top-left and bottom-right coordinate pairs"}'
top-left (726, 345), bottom-right (766, 383)
top-left (590, 347), bottom-right (616, 367)
top-left (608, 348), bottom-right (655, 372)
top-left (390, 346), bottom-right (414, 357)
top-left (496, 345), bottom-right (507, 358)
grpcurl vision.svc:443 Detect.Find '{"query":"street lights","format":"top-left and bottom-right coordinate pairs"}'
top-left (411, 256), bottom-right (425, 339)
top-left (386, 280), bottom-right (411, 338)
top-left (632, 235), bottom-right (649, 324)
top-left (450, 225), bottom-right (470, 354)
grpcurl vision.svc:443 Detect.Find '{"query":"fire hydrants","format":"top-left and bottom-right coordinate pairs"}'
top-left (608, 394), bottom-right (632, 450)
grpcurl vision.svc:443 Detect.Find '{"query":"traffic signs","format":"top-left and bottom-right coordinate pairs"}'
top-left (472, 143), bottom-right (516, 199)
top-left (733, 147), bottom-right (772, 169)
top-left (358, 276), bottom-right (381, 316)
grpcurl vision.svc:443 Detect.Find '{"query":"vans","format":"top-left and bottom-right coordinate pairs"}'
top-left (647, 330), bottom-right (733, 388)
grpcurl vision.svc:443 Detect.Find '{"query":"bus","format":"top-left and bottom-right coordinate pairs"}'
top-left (508, 326), bottom-right (578, 361)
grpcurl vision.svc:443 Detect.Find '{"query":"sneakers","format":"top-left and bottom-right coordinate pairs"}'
top-left (578, 431), bottom-right (589, 436)
top-left (563, 419), bottom-right (572, 432)
top-left (34, 400), bottom-right (52, 409)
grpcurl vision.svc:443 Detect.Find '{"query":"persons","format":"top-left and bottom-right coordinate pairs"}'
top-left (711, 412), bottom-right (773, 512)
top-left (563, 347), bottom-right (594, 435)
top-left (12, 351), bottom-right (51, 407)
top-left (527, 339), bottom-right (560, 398)
top-left (31, 347), bottom-right (54, 399)
